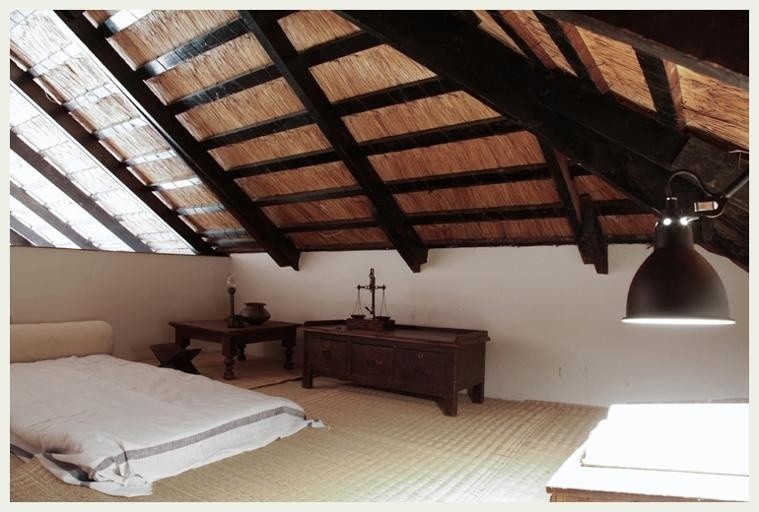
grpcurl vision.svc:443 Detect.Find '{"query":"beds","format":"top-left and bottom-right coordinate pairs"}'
top-left (10, 320), bottom-right (325, 497)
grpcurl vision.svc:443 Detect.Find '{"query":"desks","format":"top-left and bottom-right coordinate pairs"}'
top-left (302, 319), bottom-right (490, 416)
top-left (168, 319), bottom-right (299, 380)
top-left (546, 402), bottom-right (749, 502)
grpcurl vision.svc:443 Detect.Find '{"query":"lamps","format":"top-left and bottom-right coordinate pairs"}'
top-left (621, 171), bottom-right (736, 326)
